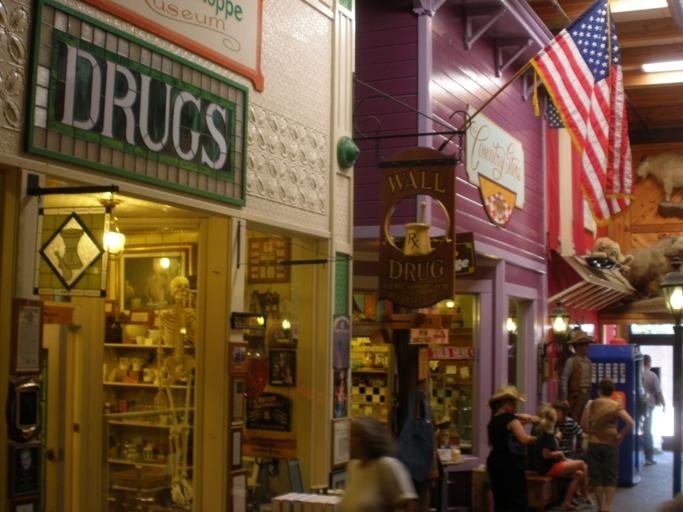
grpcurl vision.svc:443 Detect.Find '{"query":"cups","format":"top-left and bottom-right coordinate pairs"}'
top-left (119, 358), bottom-right (154, 383)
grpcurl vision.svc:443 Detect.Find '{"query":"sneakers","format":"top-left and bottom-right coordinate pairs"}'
top-left (559, 492), bottom-right (595, 511)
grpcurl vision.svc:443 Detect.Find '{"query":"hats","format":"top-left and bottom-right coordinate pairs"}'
top-left (489, 385), bottom-right (526, 404)
top-left (567, 331), bottom-right (594, 344)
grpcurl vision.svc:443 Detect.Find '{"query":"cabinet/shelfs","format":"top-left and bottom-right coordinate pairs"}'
top-left (105, 341), bottom-right (193, 470)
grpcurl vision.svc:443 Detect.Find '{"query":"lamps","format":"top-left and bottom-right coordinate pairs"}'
top-left (537, 299), bottom-right (570, 371)
top-left (101, 213), bottom-right (127, 256)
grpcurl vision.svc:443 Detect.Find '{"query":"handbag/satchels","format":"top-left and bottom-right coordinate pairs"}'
top-left (589, 445), bottom-right (613, 481)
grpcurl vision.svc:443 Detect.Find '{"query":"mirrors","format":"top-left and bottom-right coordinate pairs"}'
top-left (116, 242), bottom-right (197, 315)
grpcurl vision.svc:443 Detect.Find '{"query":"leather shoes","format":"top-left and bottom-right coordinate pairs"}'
top-left (646, 460), bottom-right (656, 465)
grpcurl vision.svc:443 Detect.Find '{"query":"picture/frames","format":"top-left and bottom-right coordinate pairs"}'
top-left (269, 347), bottom-right (296, 388)
top-left (34, 209), bottom-right (108, 299)
top-left (0, 298), bottom-right (41, 512)
top-left (329, 366), bottom-right (352, 492)
top-left (229, 343), bottom-right (249, 512)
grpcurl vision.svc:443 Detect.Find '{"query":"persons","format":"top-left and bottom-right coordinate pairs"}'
top-left (487, 380), bottom-right (634, 512)
top-left (639, 355), bottom-right (665, 465)
top-left (336, 417), bottom-right (419, 512)
top-left (560, 332), bottom-right (595, 453)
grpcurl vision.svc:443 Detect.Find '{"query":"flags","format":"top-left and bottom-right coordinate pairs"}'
top-left (530, 0), bottom-right (633, 228)
